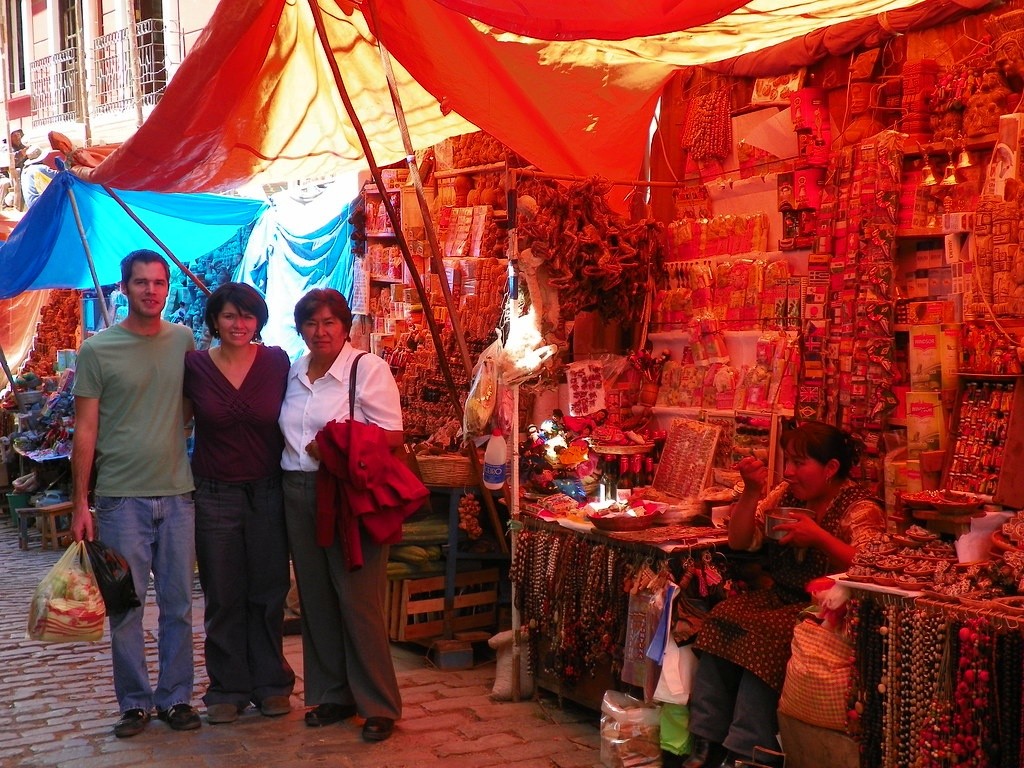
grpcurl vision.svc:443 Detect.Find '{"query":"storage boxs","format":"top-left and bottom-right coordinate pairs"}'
top-left (896, 111), bottom-right (1024, 349)
top-left (906, 390), bottom-right (956, 451)
top-left (908, 322), bottom-right (962, 392)
top-left (939, 378), bottom-right (1024, 509)
top-left (369, 137), bottom-right (505, 368)
top-left (884, 462), bottom-right (907, 516)
top-left (704, 172), bottom-right (782, 251)
top-left (906, 460), bottom-right (940, 491)
top-left (685, 107), bottom-right (780, 180)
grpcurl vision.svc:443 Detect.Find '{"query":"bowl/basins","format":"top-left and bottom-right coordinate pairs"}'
top-left (764, 506), bottom-right (817, 540)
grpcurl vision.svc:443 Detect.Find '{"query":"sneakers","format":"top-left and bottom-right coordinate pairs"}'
top-left (115, 708), bottom-right (151, 737)
top-left (158, 704), bottom-right (201, 730)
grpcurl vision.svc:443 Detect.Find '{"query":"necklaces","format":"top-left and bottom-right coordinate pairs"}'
top-left (509, 528), bottom-right (628, 682)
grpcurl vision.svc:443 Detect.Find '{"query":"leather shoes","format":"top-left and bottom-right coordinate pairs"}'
top-left (305, 703), bottom-right (356, 725)
top-left (362, 716), bottom-right (394, 739)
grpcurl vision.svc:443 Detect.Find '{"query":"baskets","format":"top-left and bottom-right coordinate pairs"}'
top-left (416, 456), bottom-right (485, 486)
top-left (586, 510), bottom-right (659, 531)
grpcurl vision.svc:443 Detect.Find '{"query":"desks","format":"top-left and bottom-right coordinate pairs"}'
top-left (520, 516), bottom-right (743, 712)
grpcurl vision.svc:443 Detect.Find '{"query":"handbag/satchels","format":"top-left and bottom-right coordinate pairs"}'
top-left (402, 452), bottom-right (432, 523)
top-left (80, 538), bottom-right (141, 616)
top-left (25, 541), bottom-right (106, 642)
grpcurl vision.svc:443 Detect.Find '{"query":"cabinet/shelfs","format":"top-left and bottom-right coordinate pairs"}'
top-left (893, 132), bottom-right (999, 335)
top-left (364, 186), bottom-right (436, 333)
top-left (647, 249), bottom-right (797, 418)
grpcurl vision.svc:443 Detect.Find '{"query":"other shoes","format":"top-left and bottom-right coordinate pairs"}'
top-left (260, 696), bottom-right (290, 714)
top-left (681, 741), bottom-right (727, 768)
top-left (721, 751), bottom-right (744, 768)
top-left (206, 703), bottom-right (238, 722)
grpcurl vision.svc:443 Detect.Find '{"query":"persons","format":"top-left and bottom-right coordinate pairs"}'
top-left (527, 408), bottom-right (608, 445)
top-left (279, 288), bottom-right (405, 742)
top-left (686, 422), bottom-right (887, 768)
top-left (0, 128), bottom-right (42, 178)
top-left (69, 249), bottom-right (198, 737)
top-left (181, 282), bottom-right (291, 724)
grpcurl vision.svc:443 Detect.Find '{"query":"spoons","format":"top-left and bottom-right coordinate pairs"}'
top-left (762, 466), bottom-right (797, 485)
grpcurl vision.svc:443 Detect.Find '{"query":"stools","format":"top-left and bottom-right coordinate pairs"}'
top-left (17, 502), bottom-right (73, 551)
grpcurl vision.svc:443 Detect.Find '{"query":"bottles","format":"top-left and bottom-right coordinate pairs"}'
top-left (482, 428), bottom-right (508, 490)
top-left (697, 410), bottom-right (708, 423)
top-left (597, 454), bottom-right (654, 502)
top-left (644, 429), bottom-right (668, 463)
